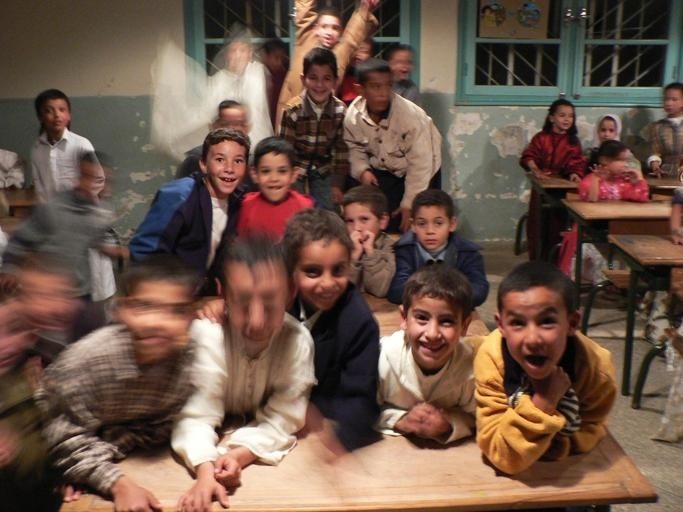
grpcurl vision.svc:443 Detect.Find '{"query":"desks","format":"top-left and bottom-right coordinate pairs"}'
top-left (527, 171), bottom-right (682, 396)
top-left (193, 292), bottom-right (493, 339)
top-left (59, 423), bottom-right (658, 512)
